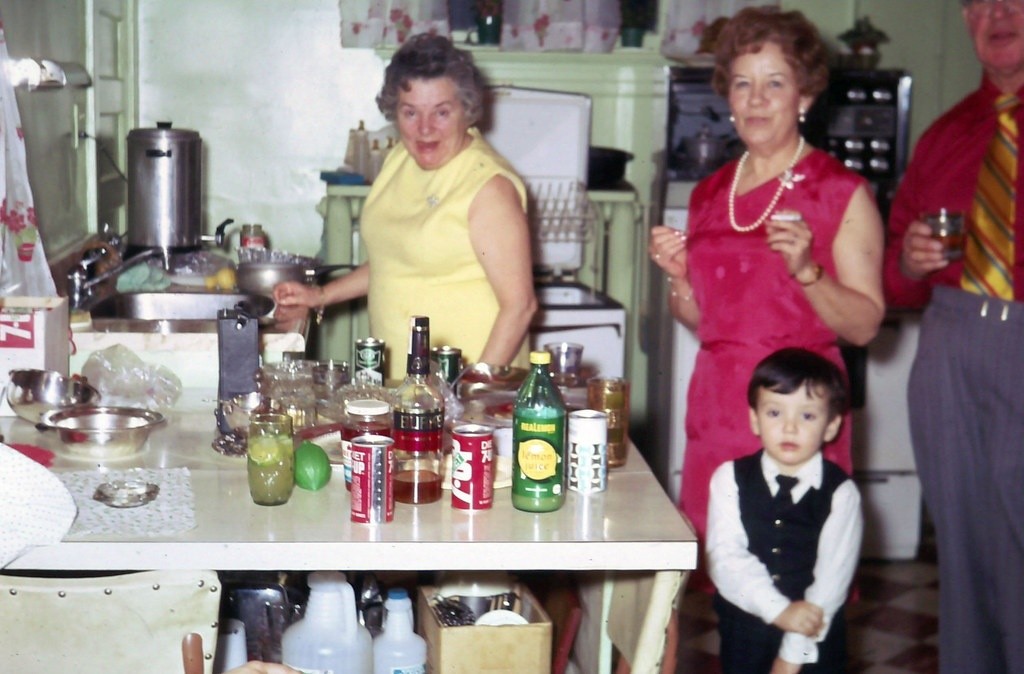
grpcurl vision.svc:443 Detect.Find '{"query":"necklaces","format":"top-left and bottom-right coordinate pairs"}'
top-left (729, 135), bottom-right (804, 231)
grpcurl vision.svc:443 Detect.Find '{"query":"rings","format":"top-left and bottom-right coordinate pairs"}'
top-left (794, 233), bottom-right (798, 243)
top-left (654, 250), bottom-right (659, 262)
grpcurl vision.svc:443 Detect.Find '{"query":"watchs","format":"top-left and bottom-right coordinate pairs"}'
top-left (794, 263), bottom-right (822, 288)
top-left (475, 362), bottom-right (491, 377)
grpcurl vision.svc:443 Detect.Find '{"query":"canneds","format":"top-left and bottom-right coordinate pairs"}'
top-left (431, 346), bottom-right (464, 399)
top-left (240, 223), bottom-right (265, 250)
top-left (567, 409), bottom-right (608, 493)
top-left (351, 435), bottom-right (395, 524)
top-left (340, 398), bottom-right (393, 491)
top-left (353, 336), bottom-right (385, 388)
top-left (450, 423), bottom-right (495, 512)
top-left (587, 376), bottom-right (625, 468)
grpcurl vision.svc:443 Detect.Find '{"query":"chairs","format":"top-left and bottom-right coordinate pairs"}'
top-left (0, 567), bottom-right (226, 674)
top-left (69, 320), bottom-right (223, 405)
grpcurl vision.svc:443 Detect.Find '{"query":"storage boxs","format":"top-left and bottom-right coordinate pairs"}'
top-left (0, 297), bottom-right (68, 385)
top-left (416, 582), bottom-right (552, 674)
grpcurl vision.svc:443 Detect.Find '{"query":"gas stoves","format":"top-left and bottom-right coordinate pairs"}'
top-left (661, 64), bottom-right (914, 223)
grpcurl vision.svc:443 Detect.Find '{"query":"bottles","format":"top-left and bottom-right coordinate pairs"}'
top-left (372, 587), bottom-right (426, 674)
top-left (241, 225), bottom-right (267, 249)
top-left (353, 121), bottom-right (395, 183)
top-left (280, 569), bottom-right (374, 674)
top-left (511, 351), bottom-right (566, 513)
top-left (392, 315), bottom-right (442, 506)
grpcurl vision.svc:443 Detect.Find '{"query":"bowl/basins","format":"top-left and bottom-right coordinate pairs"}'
top-left (6, 369), bottom-right (165, 457)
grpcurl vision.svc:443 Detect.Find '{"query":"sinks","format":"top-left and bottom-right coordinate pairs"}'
top-left (86, 291), bottom-right (250, 319)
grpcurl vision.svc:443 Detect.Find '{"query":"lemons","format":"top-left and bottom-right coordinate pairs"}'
top-left (292, 439), bottom-right (330, 491)
top-left (206, 265), bottom-right (236, 290)
top-left (248, 432), bottom-right (293, 465)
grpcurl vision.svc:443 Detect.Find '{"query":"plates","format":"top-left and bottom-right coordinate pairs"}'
top-left (473, 609), bottom-right (528, 626)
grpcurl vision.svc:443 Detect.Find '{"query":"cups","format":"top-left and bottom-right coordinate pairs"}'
top-left (920, 206), bottom-right (968, 262)
top-left (216, 358), bottom-right (396, 507)
top-left (587, 376), bottom-right (631, 469)
top-left (543, 343), bottom-right (584, 387)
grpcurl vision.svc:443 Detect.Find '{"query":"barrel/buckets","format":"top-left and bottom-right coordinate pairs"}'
top-left (128, 121), bottom-right (201, 255)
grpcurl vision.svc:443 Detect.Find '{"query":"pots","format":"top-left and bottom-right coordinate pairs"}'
top-left (238, 264), bottom-right (357, 296)
top-left (681, 133), bottom-right (739, 163)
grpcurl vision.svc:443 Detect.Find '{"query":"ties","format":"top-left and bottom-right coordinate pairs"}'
top-left (959, 92), bottom-right (1018, 302)
top-left (773, 474), bottom-right (799, 521)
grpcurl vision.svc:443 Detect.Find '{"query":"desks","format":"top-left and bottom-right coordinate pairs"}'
top-left (3, 407), bottom-right (698, 674)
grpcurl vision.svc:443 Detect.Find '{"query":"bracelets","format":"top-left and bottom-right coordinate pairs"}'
top-left (315, 283), bottom-right (325, 324)
top-left (669, 274), bottom-right (694, 300)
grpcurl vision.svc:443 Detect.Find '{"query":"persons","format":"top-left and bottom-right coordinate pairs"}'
top-left (705, 348), bottom-right (861, 674)
top-left (880, 0), bottom-right (1024, 674)
top-left (650, 4), bottom-right (885, 674)
top-left (273, 32), bottom-right (536, 381)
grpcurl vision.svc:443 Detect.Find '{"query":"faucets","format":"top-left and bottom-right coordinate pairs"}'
top-left (66, 245), bottom-right (173, 303)
